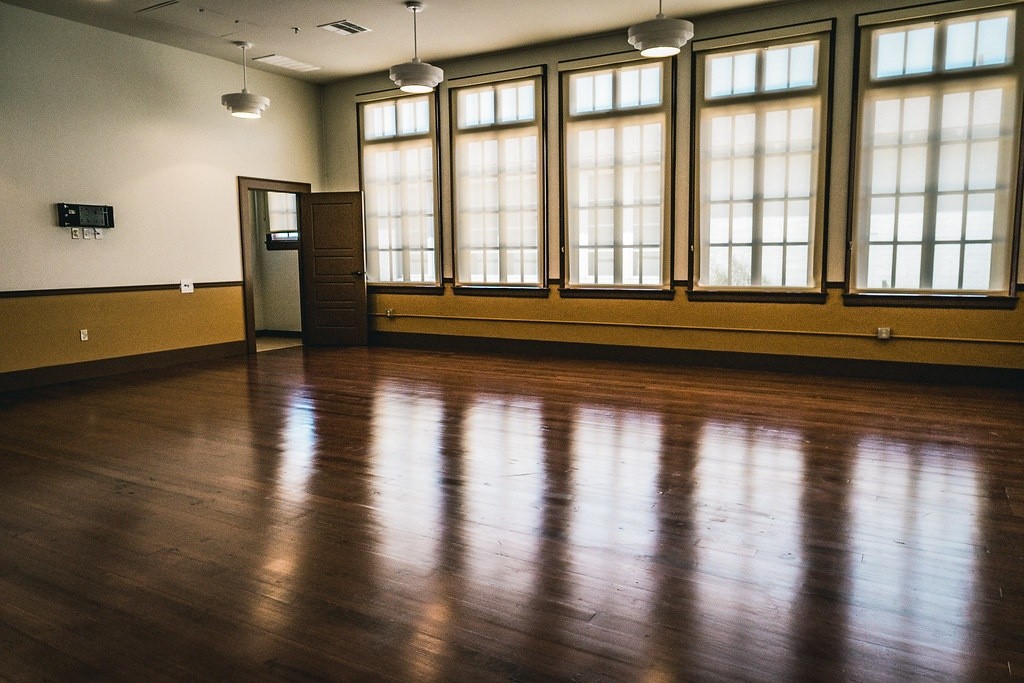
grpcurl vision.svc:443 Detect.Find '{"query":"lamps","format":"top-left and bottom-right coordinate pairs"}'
top-left (627, 0), bottom-right (695, 59)
top-left (390, 1), bottom-right (443, 95)
top-left (221, 41), bottom-right (269, 121)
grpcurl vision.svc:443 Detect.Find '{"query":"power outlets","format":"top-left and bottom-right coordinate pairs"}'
top-left (386, 308), bottom-right (396, 319)
top-left (877, 326), bottom-right (891, 340)
top-left (72, 228), bottom-right (81, 238)
top-left (81, 328), bottom-right (87, 341)
top-left (96, 229), bottom-right (103, 239)
top-left (182, 280), bottom-right (194, 293)
top-left (83, 228), bottom-right (91, 241)
